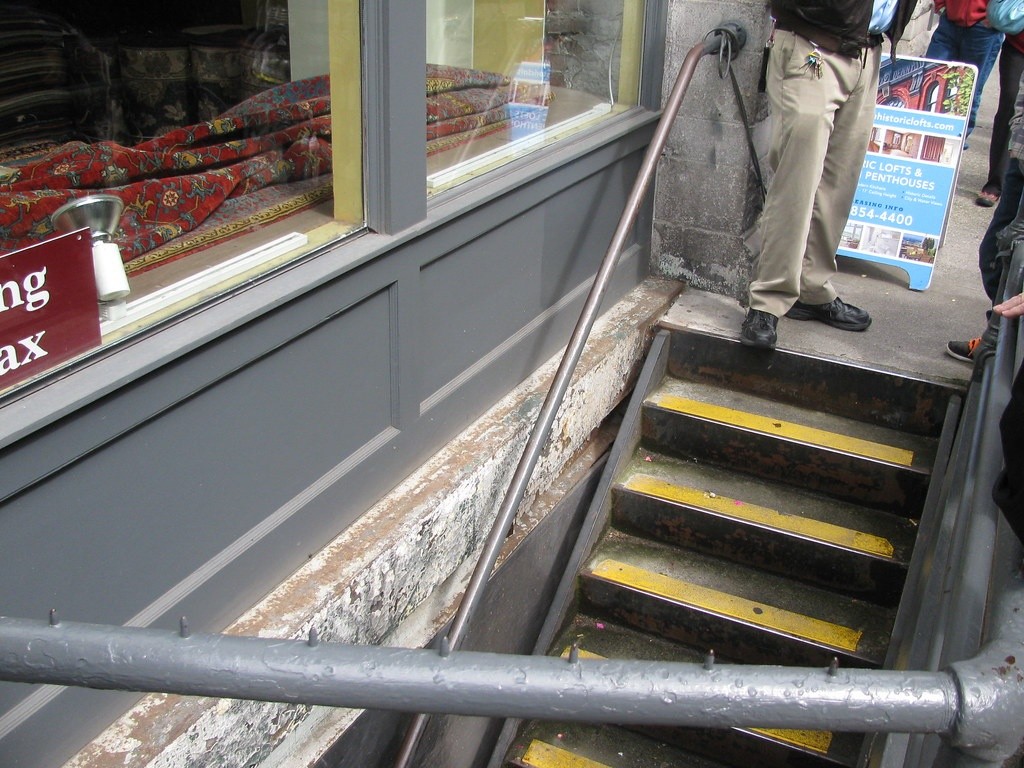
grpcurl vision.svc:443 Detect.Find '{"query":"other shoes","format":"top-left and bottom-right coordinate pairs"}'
top-left (963, 143), bottom-right (969, 150)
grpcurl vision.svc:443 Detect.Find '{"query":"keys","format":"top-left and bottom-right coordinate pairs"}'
top-left (799, 57), bottom-right (823, 79)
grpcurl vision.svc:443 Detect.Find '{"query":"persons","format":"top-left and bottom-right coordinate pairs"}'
top-left (976, 0), bottom-right (1024, 207)
top-left (993, 291), bottom-right (1024, 546)
top-left (945, 69), bottom-right (1024, 362)
top-left (740, 0), bottom-right (919, 352)
top-left (924, 0), bottom-right (1004, 149)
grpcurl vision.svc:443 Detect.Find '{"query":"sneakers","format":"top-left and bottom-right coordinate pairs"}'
top-left (976, 191), bottom-right (1000, 206)
top-left (946, 336), bottom-right (984, 364)
top-left (785, 296), bottom-right (872, 331)
top-left (740, 307), bottom-right (780, 352)
